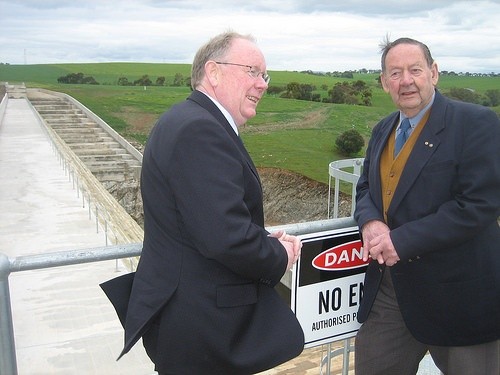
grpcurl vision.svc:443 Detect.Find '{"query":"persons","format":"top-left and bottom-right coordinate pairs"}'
top-left (99, 27), bottom-right (305, 374)
top-left (355, 32), bottom-right (500, 375)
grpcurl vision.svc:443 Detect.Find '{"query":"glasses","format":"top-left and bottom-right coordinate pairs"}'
top-left (215, 61), bottom-right (270, 84)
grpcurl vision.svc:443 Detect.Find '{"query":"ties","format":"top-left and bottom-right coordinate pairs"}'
top-left (394, 119), bottom-right (412, 159)
